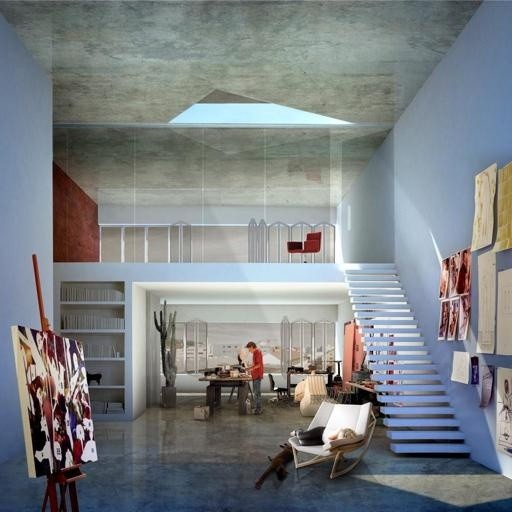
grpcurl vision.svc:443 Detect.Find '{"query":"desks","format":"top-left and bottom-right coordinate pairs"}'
top-left (198, 375), bottom-right (254, 416)
top-left (287, 372), bottom-right (332, 397)
top-left (345, 379), bottom-right (388, 428)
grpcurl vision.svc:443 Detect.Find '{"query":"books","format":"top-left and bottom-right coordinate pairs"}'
top-left (91, 399), bottom-right (125, 415)
top-left (61, 310), bottom-right (124, 329)
top-left (82, 342), bottom-right (120, 357)
top-left (60, 287), bottom-right (124, 301)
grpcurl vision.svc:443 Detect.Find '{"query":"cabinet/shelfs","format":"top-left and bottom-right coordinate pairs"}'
top-left (341, 324), bottom-right (369, 392)
top-left (53, 281), bottom-right (147, 421)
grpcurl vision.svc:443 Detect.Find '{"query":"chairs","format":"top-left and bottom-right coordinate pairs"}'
top-left (288, 399), bottom-right (377, 480)
top-left (287, 232), bottom-right (322, 263)
top-left (268, 373), bottom-right (289, 406)
top-left (299, 375), bottom-right (339, 416)
top-left (294, 370), bottom-right (315, 403)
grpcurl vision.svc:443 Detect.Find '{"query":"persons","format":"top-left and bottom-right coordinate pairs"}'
top-left (439, 299), bottom-right (471, 337)
top-left (472, 365), bottom-right (479, 384)
top-left (440, 258), bottom-right (449, 297)
top-left (288, 427), bottom-right (359, 447)
top-left (449, 257), bottom-right (457, 294)
top-left (456, 251), bottom-right (469, 294)
top-left (498, 379), bottom-right (510, 419)
top-left (240, 341), bottom-right (264, 415)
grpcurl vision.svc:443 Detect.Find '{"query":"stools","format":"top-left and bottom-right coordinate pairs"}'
top-left (338, 390), bottom-right (354, 403)
